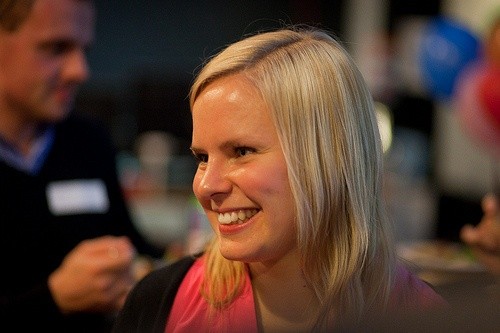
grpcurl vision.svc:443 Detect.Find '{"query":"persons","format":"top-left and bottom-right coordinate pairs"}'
top-left (0, 0), bottom-right (203, 333)
top-left (111, 29), bottom-right (455, 333)
